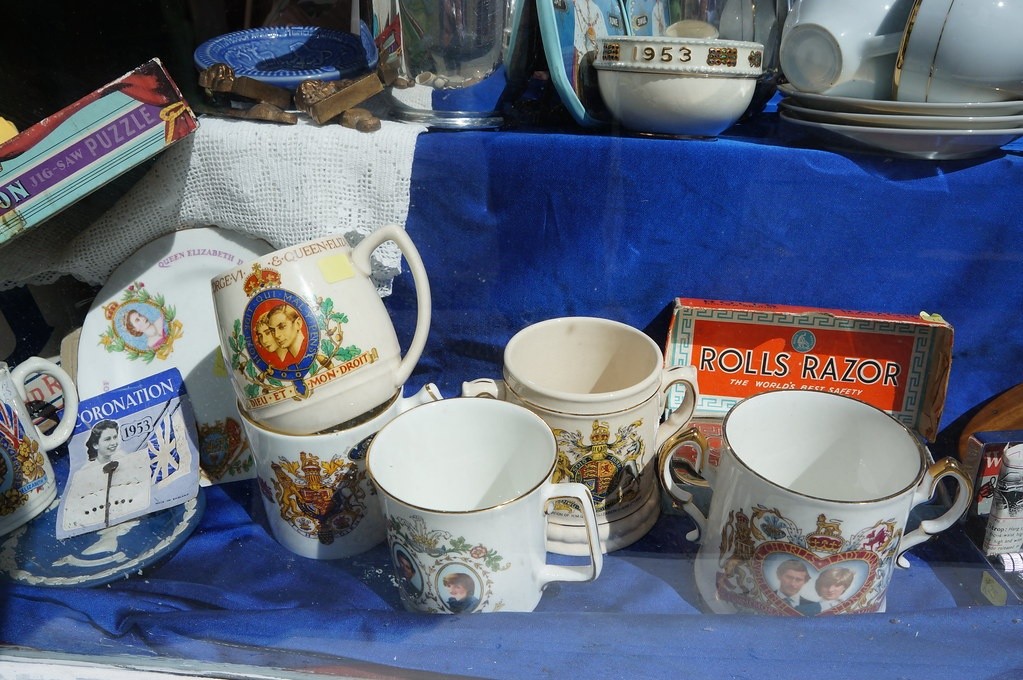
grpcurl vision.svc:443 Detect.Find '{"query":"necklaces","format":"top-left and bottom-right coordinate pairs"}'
top-left (579, 8), bottom-right (599, 36)
top-left (654, 12), bottom-right (663, 26)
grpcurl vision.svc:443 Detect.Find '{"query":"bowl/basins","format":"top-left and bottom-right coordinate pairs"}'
top-left (591, 30), bottom-right (765, 142)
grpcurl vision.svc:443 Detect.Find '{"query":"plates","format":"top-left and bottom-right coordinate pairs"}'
top-left (77, 229), bottom-right (281, 486)
top-left (774, 84), bottom-right (1023, 160)
top-left (0, 455), bottom-right (207, 588)
top-left (532, 0), bottom-right (726, 131)
top-left (196, 26), bottom-right (365, 91)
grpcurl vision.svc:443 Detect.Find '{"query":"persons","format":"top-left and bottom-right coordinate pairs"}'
top-left (121, 309), bottom-right (167, 351)
top-left (443, 572), bottom-right (478, 613)
top-left (256, 304), bottom-right (305, 362)
top-left (771, 558), bottom-right (855, 618)
top-left (396, 549), bottom-right (420, 599)
top-left (80, 419), bottom-right (127, 470)
top-left (652, 0), bottom-right (665, 37)
top-left (573, 0), bottom-right (609, 65)
top-left (440, 0), bottom-right (464, 76)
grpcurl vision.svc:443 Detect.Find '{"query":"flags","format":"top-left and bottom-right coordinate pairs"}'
top-left (149, 413), bottom-right (180, 486)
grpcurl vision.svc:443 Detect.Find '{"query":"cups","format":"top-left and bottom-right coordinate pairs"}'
top-left (656, 389), bottom-right (974, 615)
top-left (211, 226), bottom-right (432, 436)
top-left (365, 397), bottom-right (605, 614)
top-left (465, 318), bottom-right (699, 556)
top-left (0, 357), bottom-right (78, 539)
top-left (234, 384), bottom-right (442, 561)
top-left (720, 0), bottom-right (1023, 99)
top-left (354, 0), bottom-right (524, 129)
